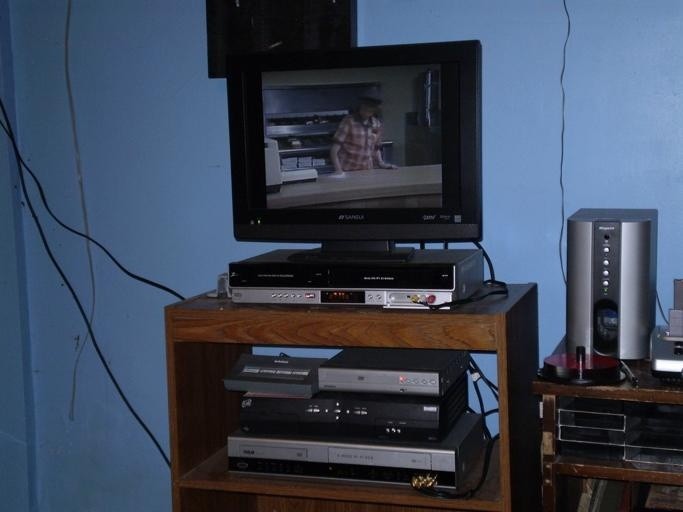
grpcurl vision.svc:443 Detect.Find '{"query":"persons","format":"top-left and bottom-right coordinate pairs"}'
top-left (325, 86), bottom-right (399, 179)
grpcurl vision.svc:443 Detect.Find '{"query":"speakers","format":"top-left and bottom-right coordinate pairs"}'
top-left (567, 209), bottom-right (658, 360)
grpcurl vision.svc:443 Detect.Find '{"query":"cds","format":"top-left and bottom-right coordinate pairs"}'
top-left (544, 354), bottom-right (621, 382)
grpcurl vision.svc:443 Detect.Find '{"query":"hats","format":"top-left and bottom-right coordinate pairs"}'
top-left (359, 86), bottom-right (385, 105)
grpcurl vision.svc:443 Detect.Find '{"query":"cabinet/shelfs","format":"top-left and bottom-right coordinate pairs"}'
top-left (531, 370), bottom-right (682, 509)
top-left (166, 282), bottom-right (541, 511)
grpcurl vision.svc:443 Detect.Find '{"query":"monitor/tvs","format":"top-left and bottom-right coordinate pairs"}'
top-left (227, 40), bottom-right (482, 264)
